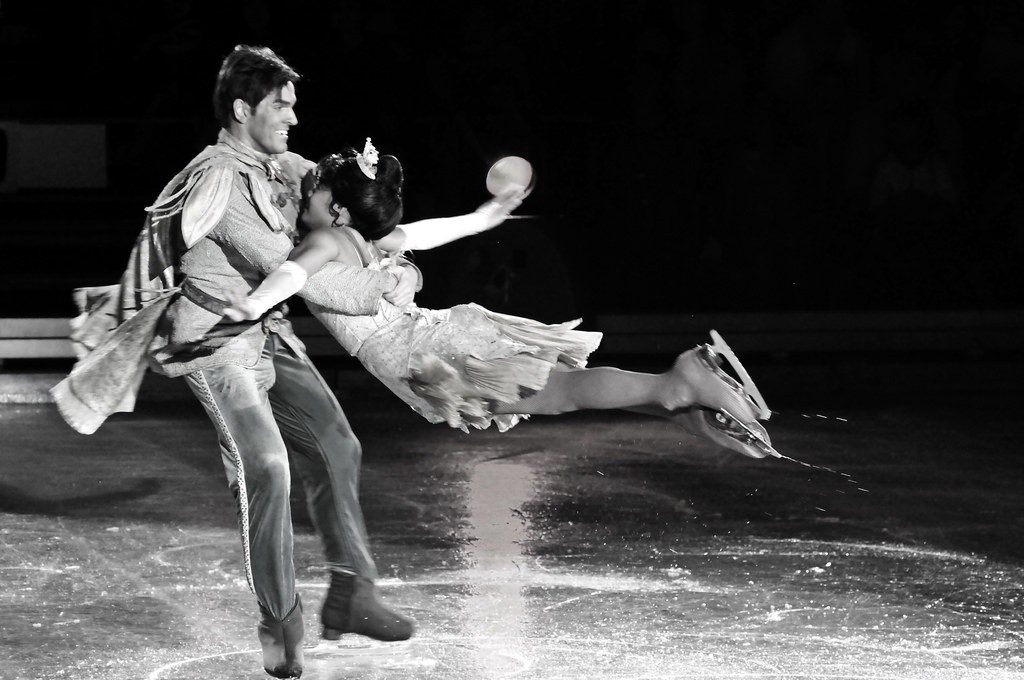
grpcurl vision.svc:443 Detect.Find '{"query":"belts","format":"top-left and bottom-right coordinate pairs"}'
top-left (180, 281), bottom-right (233, 320)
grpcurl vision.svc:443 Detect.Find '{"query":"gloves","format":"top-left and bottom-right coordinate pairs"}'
top-left (222, 260), bottom-right (308, 321)
top-left (396, 183), bottom-right (525, 252)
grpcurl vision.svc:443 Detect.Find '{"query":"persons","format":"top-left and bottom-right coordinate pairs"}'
top-left (146, 42), bottom-right (425, 680)
top-left (220, 139), bottom-right (773, 459)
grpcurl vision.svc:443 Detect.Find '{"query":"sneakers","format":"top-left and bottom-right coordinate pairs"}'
top-left (663, 406), bottom-right (781, 459)
top-left (256, 592), bottom-right (305, 680)
top-left (658, 330), bottom-right (772, 424)
top-left (318, 570), bottom-right (413, 642)
